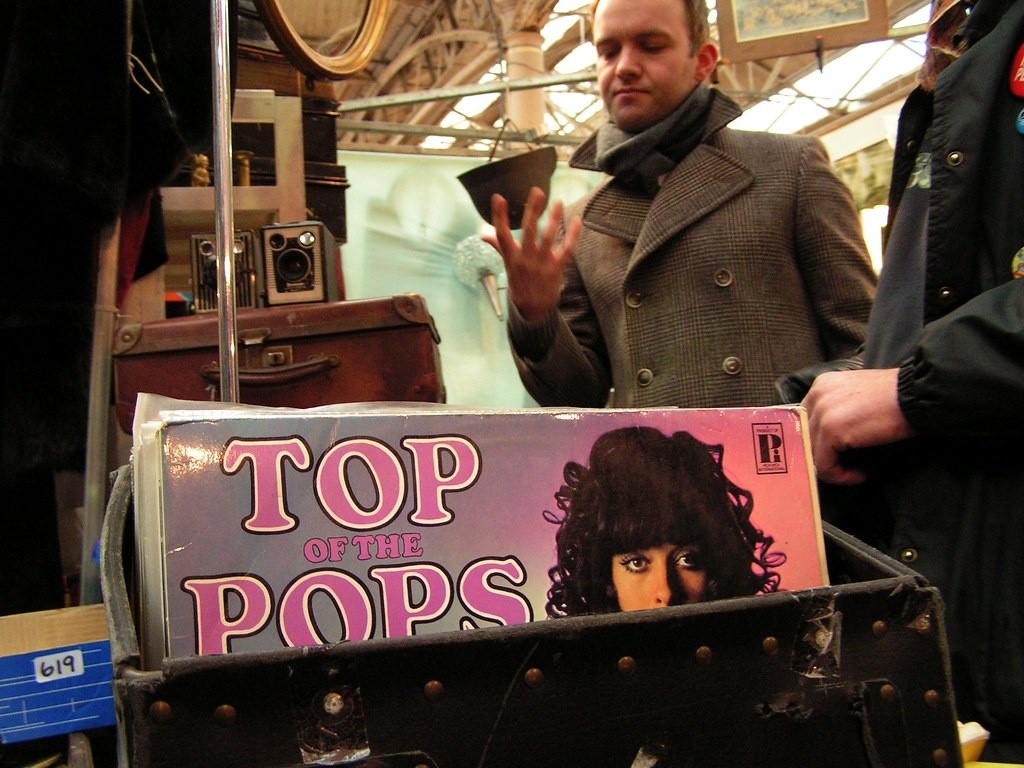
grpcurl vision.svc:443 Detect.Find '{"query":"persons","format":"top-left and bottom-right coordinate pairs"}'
top-left (543, 426), bottom-right (786, 618)
top-left (775, 1), bottom-right (1024, 764)
top-left (482, 0), bottom-right (879, 408)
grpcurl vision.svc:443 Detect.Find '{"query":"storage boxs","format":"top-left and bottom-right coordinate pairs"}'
top-left (0, 603), bottom-right (116, 744)
top-left (99, 462), bottom-right (967, 766)
top-left (165, 0), bottom-right (351, 249)
